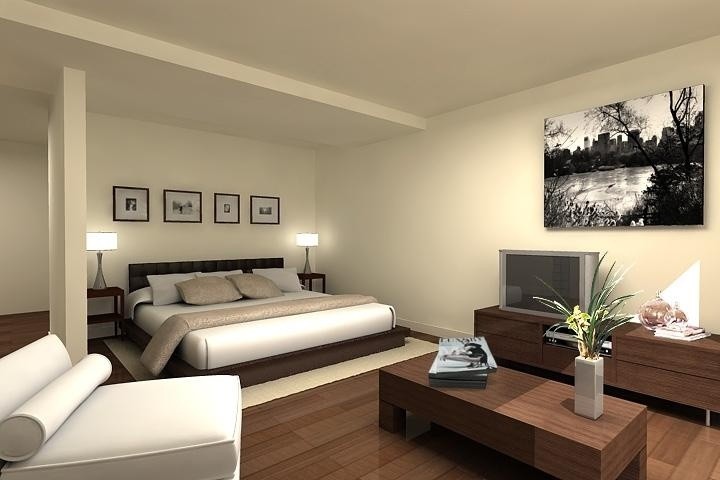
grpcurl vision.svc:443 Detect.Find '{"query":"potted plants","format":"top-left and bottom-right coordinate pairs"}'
top-left (531, 249), bottom-right (643, 421)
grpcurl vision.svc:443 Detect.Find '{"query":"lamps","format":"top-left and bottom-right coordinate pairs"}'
top-left (295, 232), bottom-right (322, 276)
top-left (84, 230), bottom-right (122, 292)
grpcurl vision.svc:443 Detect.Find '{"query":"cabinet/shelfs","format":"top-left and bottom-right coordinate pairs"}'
top-left (471, 305), bottom-right (720, 430)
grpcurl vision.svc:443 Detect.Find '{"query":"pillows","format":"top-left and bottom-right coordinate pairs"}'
top-left (193, 266), bottom-right (243, 277)
top-left (252, 266), bottom-right (303, 294)
top-left (144, 269), bottom-right (203, 308)
top-left (0, 351), bottom-right (117, 466)
top-left (224, 271), bottom-right (286, 299)
top-left (173, 274), bottom-right (245, 306)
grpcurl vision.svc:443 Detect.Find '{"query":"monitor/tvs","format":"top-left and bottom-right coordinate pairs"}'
top-left (499, 249), bottom-right (599, 320)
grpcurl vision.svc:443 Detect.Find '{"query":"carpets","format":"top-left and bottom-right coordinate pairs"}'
top-left (99, 332), bottom-right (443, 414)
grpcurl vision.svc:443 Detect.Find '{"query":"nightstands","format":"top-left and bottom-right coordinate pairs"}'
top-left (87, 286), bottom-right (127, 343)
top-left (297, 270), bottom-right (327, 294)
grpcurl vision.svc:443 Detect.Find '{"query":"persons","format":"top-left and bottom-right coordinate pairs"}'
top-left (443, 343), bottom-right (487, 368)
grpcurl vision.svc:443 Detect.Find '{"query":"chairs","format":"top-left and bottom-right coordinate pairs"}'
top-left (0, 334), bottom-right (249, 480)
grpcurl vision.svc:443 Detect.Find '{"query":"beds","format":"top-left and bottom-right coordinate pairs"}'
top-left (117, 256), bottom-right (407, 391)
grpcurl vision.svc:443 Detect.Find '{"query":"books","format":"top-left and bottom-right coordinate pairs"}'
top-left (653, 322), bottom-right (712, 342)
top-left (437, 336), bottom-right (496, 378)
top-left (430, 377), bottom-right (486, 389)
top-left (428, 350), bottom-right (488, 381)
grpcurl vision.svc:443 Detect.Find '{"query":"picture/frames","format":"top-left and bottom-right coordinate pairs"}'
top-left (214, 193), bottom-right (241, 225)
top-left (162, 188), bottom-right (203, 223)
top-left (249, 194), bottom-right (281, 226)
top-left (112, 185), bottom-right (150, 222)
